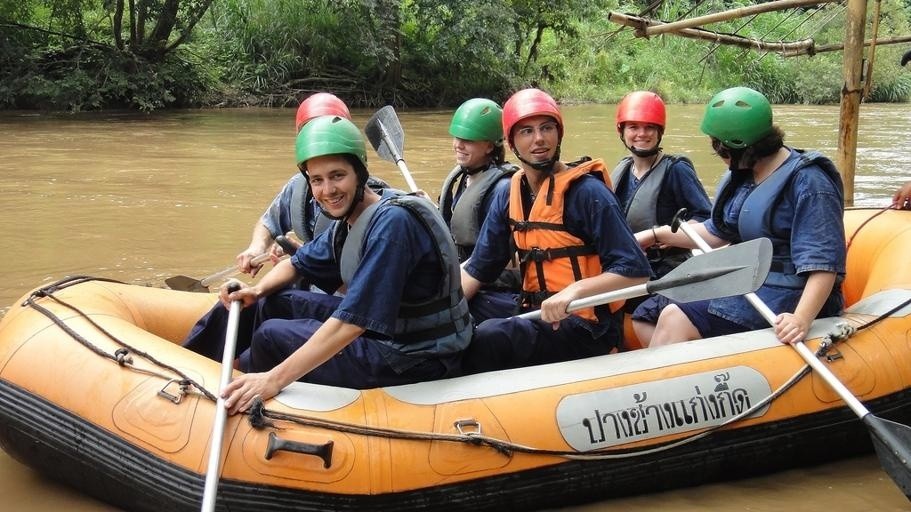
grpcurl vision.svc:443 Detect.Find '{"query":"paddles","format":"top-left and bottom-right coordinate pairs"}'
top-left (364, 105), bottom-right (418, 193)
top-left (505, 236), bottom-right (774, 322)
top-left (671, 208), bottom-right (910, 503)
top-left (164, 235), bottom-right (296, 292)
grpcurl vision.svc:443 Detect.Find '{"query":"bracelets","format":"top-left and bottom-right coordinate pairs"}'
top-left (651, 224), bottom-right (660, 245)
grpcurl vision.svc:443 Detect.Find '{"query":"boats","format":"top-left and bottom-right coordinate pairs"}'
top-left (0, 206), bottom-right (911, 512)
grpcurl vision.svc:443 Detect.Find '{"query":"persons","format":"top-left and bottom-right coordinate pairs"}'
top-left (609, 91), bottom-right (716, 317)
top-left (892, 182), bottom-right (911, 209)
top-left (460, 87), bottom-right (659, 372)
top-left (412, 97), bottom-right (521, 298)
top-left (235, 92), bottom-right (391, 299)
top-left (183, 112), bottom-right (473, 418)
top-left (616, 86), bottom-right (848, 350)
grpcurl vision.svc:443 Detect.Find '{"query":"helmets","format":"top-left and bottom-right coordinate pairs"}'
top-left (295, 92), bottom-right (369, 182)
top-left (449, 98), bottom-right (502, 147)
top-left (701, 87), bottom-right (771, 150)
top-left (501, 89), bottom-right (563, 151)
top-left (617, 91), bottom-right (666, 133)
